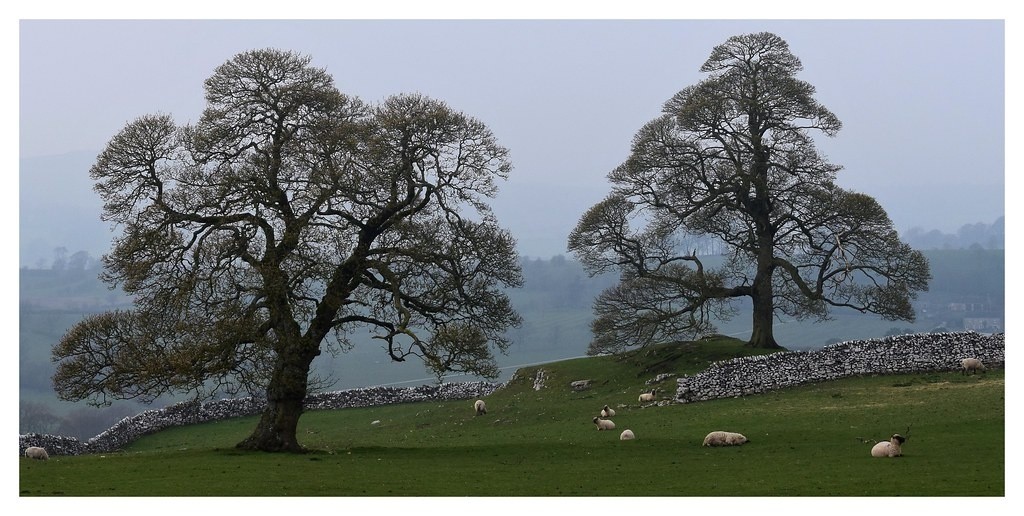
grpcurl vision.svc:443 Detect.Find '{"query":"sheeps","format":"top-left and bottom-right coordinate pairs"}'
top-left (638, 388), bottom-right (658, 401)
top-left (620, 429), bottom-right (635, 440)
top-left (600, 405), bottom-right (615, 417)
top-left (871, 434), bottom-right (906, 457)
top-left (703, 431), bottom-right (751, 447)
top-left (25, 447), bottom-right (50, 461)
top-left (475, 399), bottom-right (488, 417)
top-left (592, 416), bottom-right (616, 431)
top-left (960, 358), bottom-right (990, 377)
